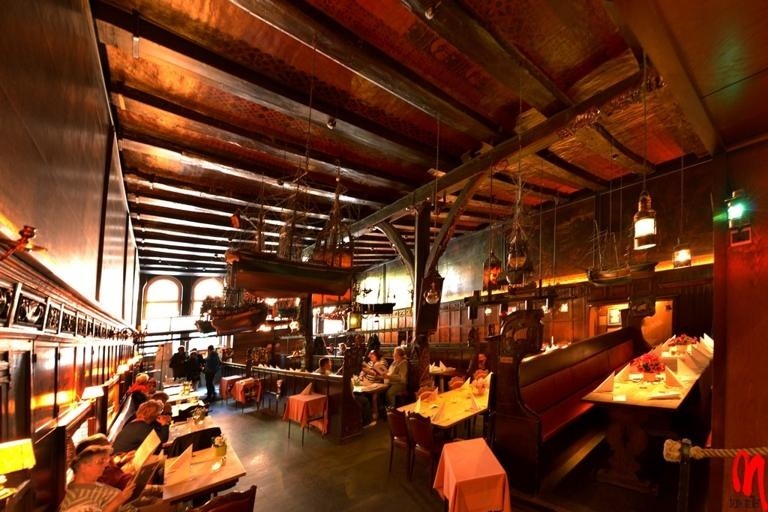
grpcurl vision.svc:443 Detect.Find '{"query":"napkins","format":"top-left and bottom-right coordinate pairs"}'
top-left (165, 443), bottom-right (193, 487)
top-left (431, 400), bottom-right (449, 424)
top-left (615, 361), bottom-right (631, 381)
top-left (467, 389), bottom-right (482, 411)
top-left (481, 371), bottom-right (493, 387)
top-left (593, 370), bottom-right (616, 393)
top-left (650, 334), bottom-right (677, 358)
top-left (676, 332), bottom-right (713, 379)
top-left (459, 375), bottom-right (472, 391)
top-left (664, 365), bottom-right (685, 388)
top-left (413, 396), bottom-right (425, 414)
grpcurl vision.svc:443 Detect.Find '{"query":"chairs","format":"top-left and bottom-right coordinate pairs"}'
top-left (386, 405), bottom-right (443, 480)
top-left (185, 484), bottom-right (258, 512)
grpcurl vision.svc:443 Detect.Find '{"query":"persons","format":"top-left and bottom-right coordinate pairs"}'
top-left (463, 352), bottom-right (491, 385)
top-left (313, 333), bottom-right (409, 422)
top-left (58, 345), bottom-right (221, 512)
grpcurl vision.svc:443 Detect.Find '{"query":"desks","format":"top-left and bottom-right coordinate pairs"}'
top-left (230, 378), bottom-right (263, 412)
top-left (218, 374), bottom-right (244, 407)
top-left (283, 393), bottom-right (329, 445)
top-left (434, 437), bottom-right (511, 512)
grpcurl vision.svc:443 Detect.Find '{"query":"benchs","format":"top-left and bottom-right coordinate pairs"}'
top-left (105, 393), bottom-right (138, 444)
top-left (515, 325), bottom-right (644, 469)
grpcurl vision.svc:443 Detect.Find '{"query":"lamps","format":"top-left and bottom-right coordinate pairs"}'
top-left (671, 138), bottom-right (693, 270)
top-left (423, 74), bottom-right (535, 305)
top-left (0, 437), bottom-right (38, 475)
top-left (631, 48), bottom-right (658, 252)
top-left (81, 385), bottom-right (105, 403)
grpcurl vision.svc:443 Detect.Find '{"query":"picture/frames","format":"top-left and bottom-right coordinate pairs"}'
top-left (607, 307), bottom-right (622, 327)
